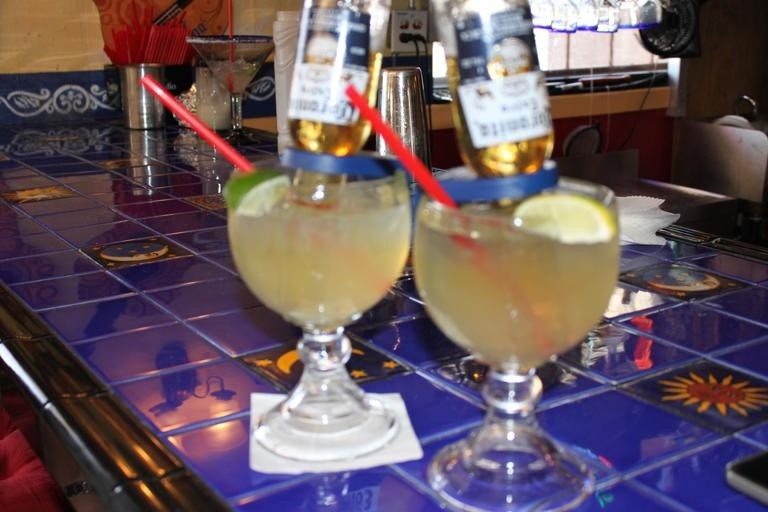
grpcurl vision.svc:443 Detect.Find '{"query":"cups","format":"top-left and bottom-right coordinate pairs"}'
top-left (195, 133), bottom-right (249, 204)
top-left (190, 65), bottom-right (233, 140)
top-left (125, 131), bottom-right (170, 197)
top-left (118, 62), bottom-right (167, 132)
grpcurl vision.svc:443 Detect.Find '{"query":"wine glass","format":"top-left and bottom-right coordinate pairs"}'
top-left (223, 148), bottom-right (412, 468)
top-left (184, 34), bottom-right (273, 152)
top-left (412, 161), bottom-right (623, 509)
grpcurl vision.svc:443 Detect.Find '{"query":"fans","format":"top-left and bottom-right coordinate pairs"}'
top-left (634, 1), bottom-right (703, 62)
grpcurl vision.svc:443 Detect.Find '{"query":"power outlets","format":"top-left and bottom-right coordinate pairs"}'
top-left (390, 9), bottom-right (428, 55)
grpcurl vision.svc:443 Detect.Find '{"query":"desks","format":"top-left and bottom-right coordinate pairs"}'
top-left (577, 175), bottom-right (741, 241)
top-left (1, 112), bottom-right (768, 512)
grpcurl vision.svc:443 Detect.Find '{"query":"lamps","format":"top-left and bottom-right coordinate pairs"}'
top-left (145, 340), bottom-right (251, 459)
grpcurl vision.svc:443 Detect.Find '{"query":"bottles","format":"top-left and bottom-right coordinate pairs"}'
top-left (426, 0), bottom-right (561, 212)
top-left (375, 62), bottom-right (435, 186)
top-left (282, 0), bottom-right (391, 214)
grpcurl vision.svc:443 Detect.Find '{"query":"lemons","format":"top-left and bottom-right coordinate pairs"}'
top-left (513, 192), bottom-right (618, 245)
top-left (220, 167), bottom-right (293, 220)
